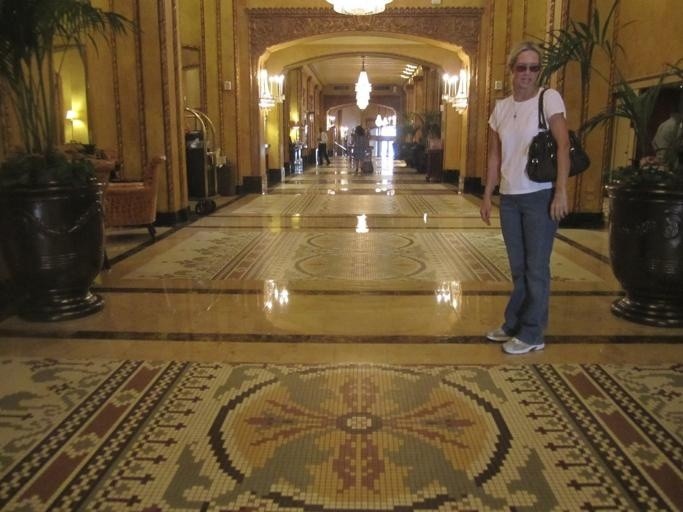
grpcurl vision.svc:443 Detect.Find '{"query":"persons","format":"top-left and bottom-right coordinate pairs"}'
top-left (316, 127), bottom-right (331, 166)
top-left (348, 125), bottom-right (370, 172)
top-left (477, 42), bottom-right (573, 355)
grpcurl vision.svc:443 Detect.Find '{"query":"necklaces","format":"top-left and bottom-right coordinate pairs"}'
top-left (511, 95), bottom-right (526, 119)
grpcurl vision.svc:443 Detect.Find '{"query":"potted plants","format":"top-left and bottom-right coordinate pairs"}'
top-left (1, 0), bottom-right (140, 326)
top-left (398, 108), bottom-right (442, 149)
top-left (530, 2), bottom-right (682, 328)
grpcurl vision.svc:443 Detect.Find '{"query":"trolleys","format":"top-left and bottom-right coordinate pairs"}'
top-left (359, 149), bottom-right (373, 175)
top-left (183, 106), bottom-right (218, 214)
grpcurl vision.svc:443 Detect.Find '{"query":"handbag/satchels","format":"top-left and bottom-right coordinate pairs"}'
top-left (526, 128), bottom-right (590, 182)
top-left (361, 161), bottom-right (373, 173)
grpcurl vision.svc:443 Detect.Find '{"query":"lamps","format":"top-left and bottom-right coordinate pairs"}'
top-left (258, 59), bottom-right (287, 119)
top-left (353, 54), bottom-right (371, 113)
top-left (439, 59), bottom-right (471, 116)
top-left (322, 0), bottom-right (399, 21)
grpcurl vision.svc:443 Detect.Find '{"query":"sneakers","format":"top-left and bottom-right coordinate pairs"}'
top-left (486, 326), bottom-right (546, 355)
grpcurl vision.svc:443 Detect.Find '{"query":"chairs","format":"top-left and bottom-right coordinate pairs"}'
top-left (98, 150), bottom-right (168, 245)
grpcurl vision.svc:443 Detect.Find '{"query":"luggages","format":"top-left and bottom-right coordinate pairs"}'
top-left (512, 63), bottom-right (540, 72)
